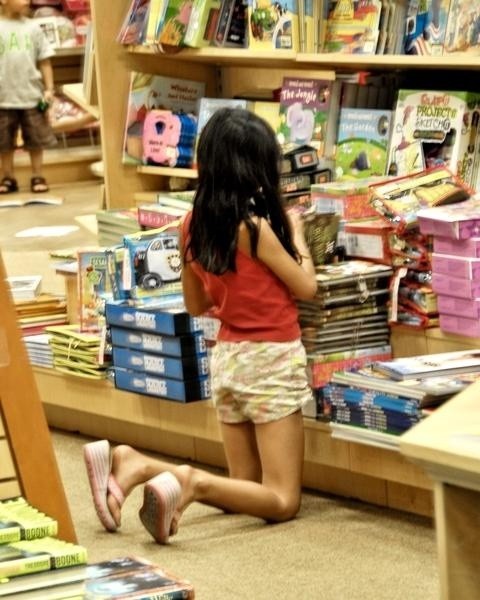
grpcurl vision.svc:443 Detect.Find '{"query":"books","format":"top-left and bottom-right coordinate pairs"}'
top-left (0, 495), bottom-right (195, 600)
top-left (119, 0), bottom-right (480, 338)
top-left (6, 219), bottom-right (478, 436)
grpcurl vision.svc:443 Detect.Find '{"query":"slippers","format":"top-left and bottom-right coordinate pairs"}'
top-left (0, 177), bottom-right (19, 194)
top-left (82, 439), bottom-right (126, 532)
top-left (138, 471), bottom-right (183, 546)
top-left (31, 177), bottom-right (48, 192)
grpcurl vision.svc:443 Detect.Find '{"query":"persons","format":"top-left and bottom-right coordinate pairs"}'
top-left (78, 109), bottom-right (318, 544)
top-left (0, 1), bottom-right (56, 193)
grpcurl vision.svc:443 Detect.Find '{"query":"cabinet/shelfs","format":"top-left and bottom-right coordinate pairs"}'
top-left (88, 1), bottom-right (480, 213)
